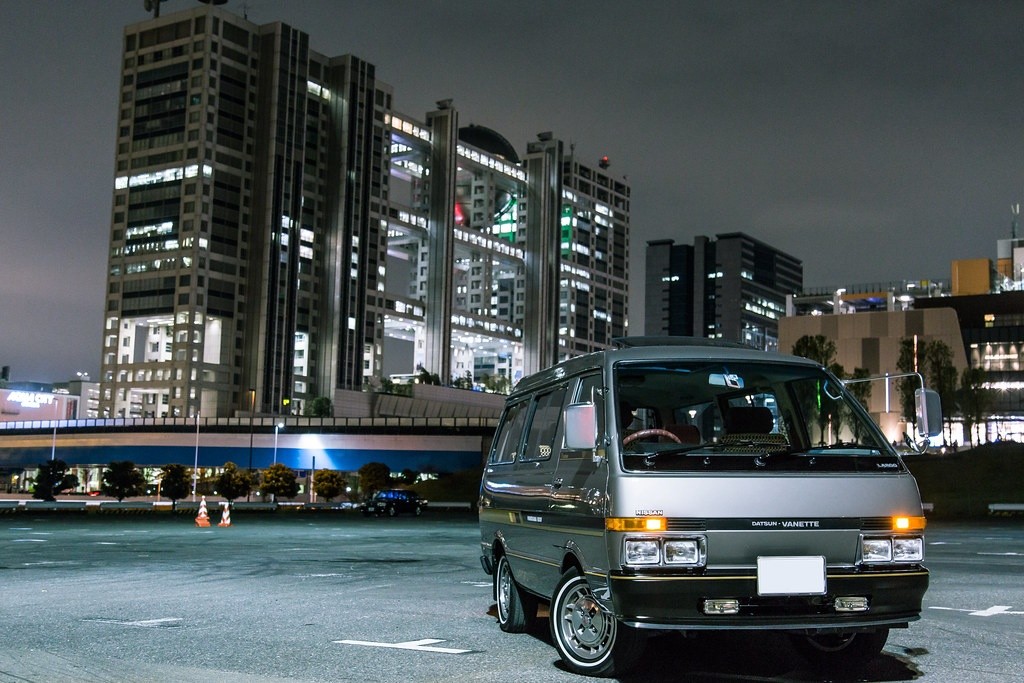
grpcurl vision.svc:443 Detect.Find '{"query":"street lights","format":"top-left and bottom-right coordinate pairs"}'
top-left (51, 397), bottom-right (58, 463)
top-left (189, 411), bottom-right (200, 504)
top-left (273, 423), bottom-right (284, 466)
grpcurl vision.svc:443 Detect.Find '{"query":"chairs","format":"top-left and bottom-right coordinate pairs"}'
top-left (721, 406), bottom-right (790, 452)
top-left (619, 400), bottom-right (638, 451)
top-left (697, 403), bottom-right (733, 442)
top-left (661, 423), bottom-right (703, 445)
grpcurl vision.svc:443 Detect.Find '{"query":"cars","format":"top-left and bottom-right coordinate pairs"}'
top-left (365, 488), bottom-right (429, 518)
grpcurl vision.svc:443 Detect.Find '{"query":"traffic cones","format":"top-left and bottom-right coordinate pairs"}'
top-left (218, 502), bottom-right (234, 528)
top-left (194, 494), bottom-right (210, 527)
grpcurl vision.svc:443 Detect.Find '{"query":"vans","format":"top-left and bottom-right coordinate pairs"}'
top-left (477, 336), bottom-right (943, 677)
